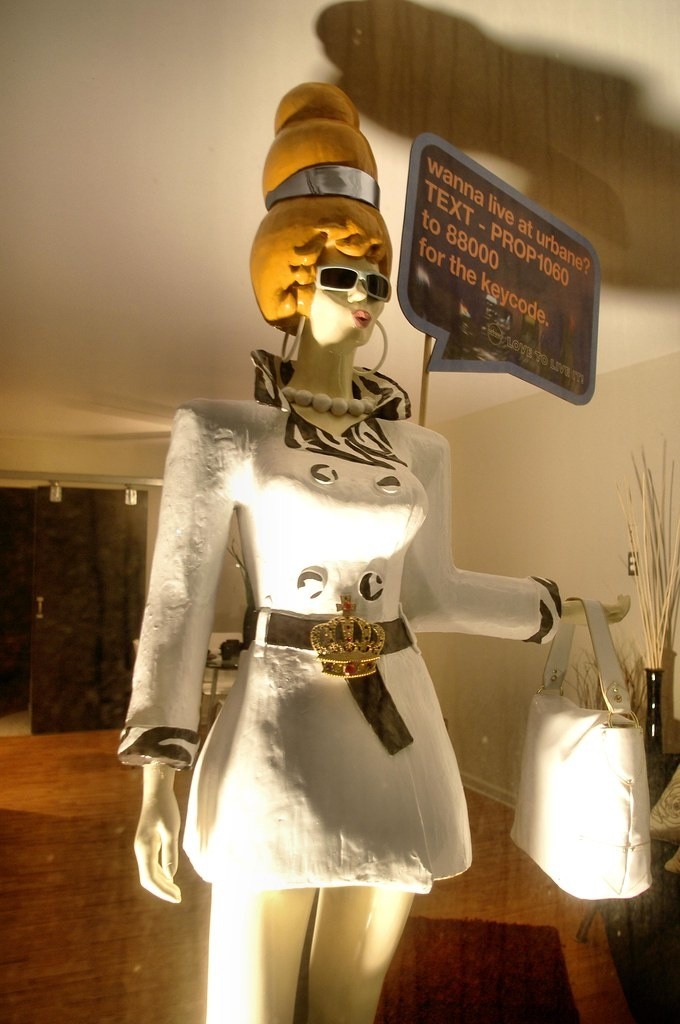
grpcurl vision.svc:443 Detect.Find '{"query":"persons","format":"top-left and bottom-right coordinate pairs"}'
top-left (117, 83), bottom-right (632, 1024)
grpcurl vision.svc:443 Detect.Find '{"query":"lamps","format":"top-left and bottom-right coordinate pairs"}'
top-left (126, 483), bottom-right (138, 506)
top-left (50, 480), bottom-right (63, 503)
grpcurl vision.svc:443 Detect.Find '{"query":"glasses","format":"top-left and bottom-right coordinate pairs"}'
top-left (315, 266), bottom-right (392, 303)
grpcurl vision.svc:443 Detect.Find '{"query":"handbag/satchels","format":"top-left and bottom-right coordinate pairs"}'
top-left (509, 597), bottom-right (653, 900)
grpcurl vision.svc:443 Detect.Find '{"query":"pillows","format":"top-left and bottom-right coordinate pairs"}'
top-left (650, 763), bottom-right (679, 873)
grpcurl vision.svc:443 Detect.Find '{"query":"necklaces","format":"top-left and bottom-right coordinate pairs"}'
top-left (283, 385), bottom-right (374, 415)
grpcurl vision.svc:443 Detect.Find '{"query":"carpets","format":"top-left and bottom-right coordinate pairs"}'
top-left (375, 916), bottom-right (582, 1024)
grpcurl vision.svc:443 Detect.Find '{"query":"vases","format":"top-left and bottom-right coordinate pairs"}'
top-left (241, 608), bottom-right (257, 643)
top-left (644, 668), bottom-right (664, 809)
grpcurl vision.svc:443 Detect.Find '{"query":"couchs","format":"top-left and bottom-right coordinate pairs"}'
top-left (577, 753), bottom-right (680, 1024)
